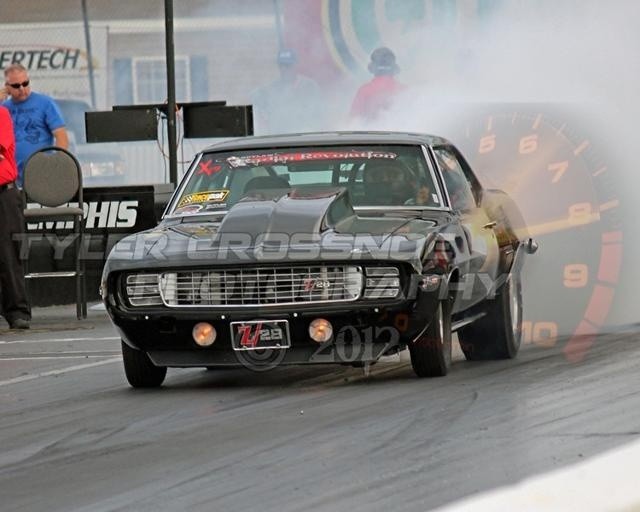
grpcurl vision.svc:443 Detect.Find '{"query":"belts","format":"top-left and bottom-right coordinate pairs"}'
top-left (0, 182), bottom-right (15, 191)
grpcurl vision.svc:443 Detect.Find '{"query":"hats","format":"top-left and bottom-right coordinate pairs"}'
top-left (277, 46), bottom-right (299, 65)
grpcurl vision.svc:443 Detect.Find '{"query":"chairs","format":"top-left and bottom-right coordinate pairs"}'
top-left (242, 176), bottom-right (290, 193)
top-left (19, 146), bottom-right (88, 320)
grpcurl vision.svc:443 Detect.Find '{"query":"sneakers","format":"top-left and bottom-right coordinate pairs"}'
top-left (10, 318), bottom-right (29, 329)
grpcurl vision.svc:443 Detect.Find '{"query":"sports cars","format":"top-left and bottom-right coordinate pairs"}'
top-left (97, 128), bottom-right (539, 391)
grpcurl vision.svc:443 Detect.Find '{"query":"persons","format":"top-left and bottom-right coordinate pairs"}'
top-left (351, 46), bottom-right (404, 121)
top-left (0, 64), bottom-right (70, 191)
top-left (257, 48), bottom-right (323, 134)
top-left (0, 105), bottom-right (32, 328)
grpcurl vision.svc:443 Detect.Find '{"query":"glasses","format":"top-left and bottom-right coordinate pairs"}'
top-left (8, 80), bottom-right (29, 88)
